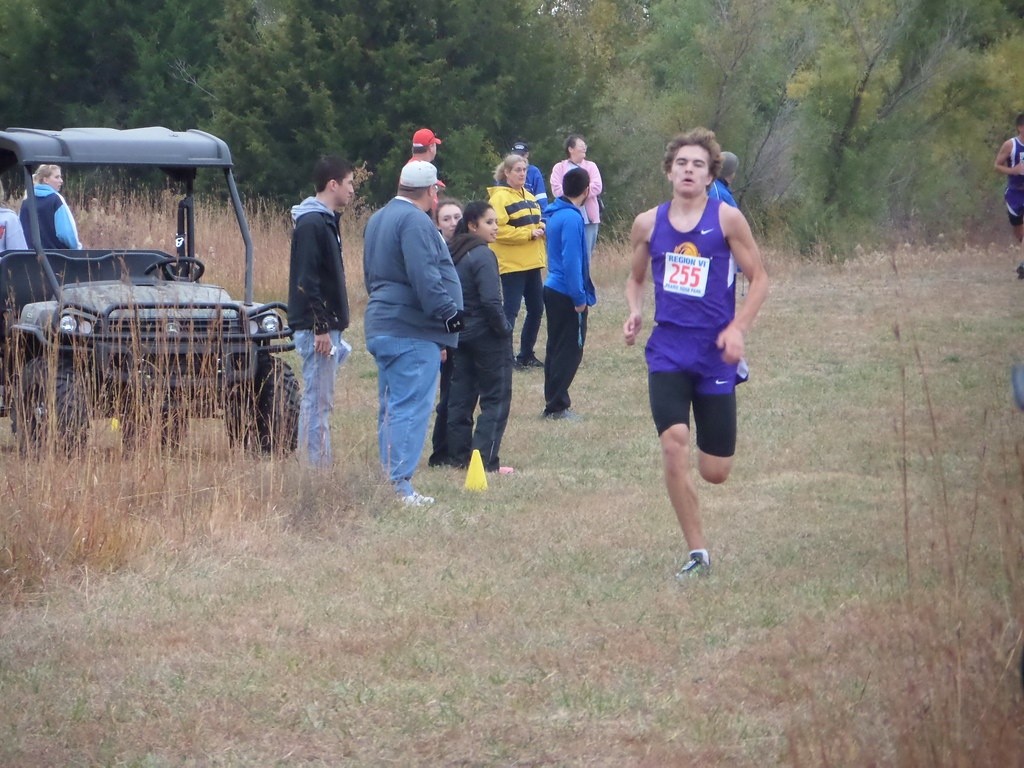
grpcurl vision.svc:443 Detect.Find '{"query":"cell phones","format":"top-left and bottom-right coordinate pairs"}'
top-left (313, 341), bottom-right (336, 356)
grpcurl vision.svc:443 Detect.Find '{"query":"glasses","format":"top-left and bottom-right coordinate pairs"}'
top-left (433, 185), bottom-right (438, 193)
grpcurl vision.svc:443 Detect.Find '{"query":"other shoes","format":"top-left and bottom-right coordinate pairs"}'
top-left (545, 408), bottom-right (584, 421)
top-left (517, 351), bottom-right (544, 368)
top-left (1016, 261), bottom-right (1024, 280)
top-left (511, 356), bottom-right (531, 373)
top-left (498, 465), bottom-right (514, 475)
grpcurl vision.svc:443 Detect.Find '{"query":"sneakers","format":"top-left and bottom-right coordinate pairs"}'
top-left (673, 549), bottom-right (712, 581)
top-left (399, 492), bottom-right (435, 507)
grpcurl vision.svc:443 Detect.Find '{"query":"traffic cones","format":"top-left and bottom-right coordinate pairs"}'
top-left (463, 449), bottom-right (490, 494)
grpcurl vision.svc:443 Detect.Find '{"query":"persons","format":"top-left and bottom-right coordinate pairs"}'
top-left (362, 160), bottom-right (465, 510)
top-left (427, 196), bottom-right (465, 467)
top-left (19, 161), bottom-right (82, 250)
top-left (443, 198), bottom-right (517, 476)
top-left (486, 152), bottom-right (548, 370)
top-left (992, 114), bottom-right (1024, 280)
top-left (405, 128), bottom-right (441, 216)
top-left (622, 123), bottom-right (769, 579)
top-left (495, 141), bottom-right (550, 223)
top-left (707, 149), bottom-right (744, 274)
top-left (284, 156), bottom-right (355, 471)
top-left (542, 167), bottom-right (597, 423)
top-left (548, 134), bottom-right (602, 266)
top-left (0, 206), bottom-right (28, 253)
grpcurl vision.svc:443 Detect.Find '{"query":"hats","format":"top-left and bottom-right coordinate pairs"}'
top-left (413, 128), bottom-right (441, 147)
top-left (512, 142), bottom-right (529, 152)
top-left (399, 160), bottom-right (447, 188)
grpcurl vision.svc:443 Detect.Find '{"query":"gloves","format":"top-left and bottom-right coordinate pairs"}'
top-left (445, 308), bottom-right (473, 334)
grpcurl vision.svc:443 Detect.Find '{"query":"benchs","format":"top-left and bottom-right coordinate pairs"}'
top-left (0, 252), bottom-right (173, 316)
